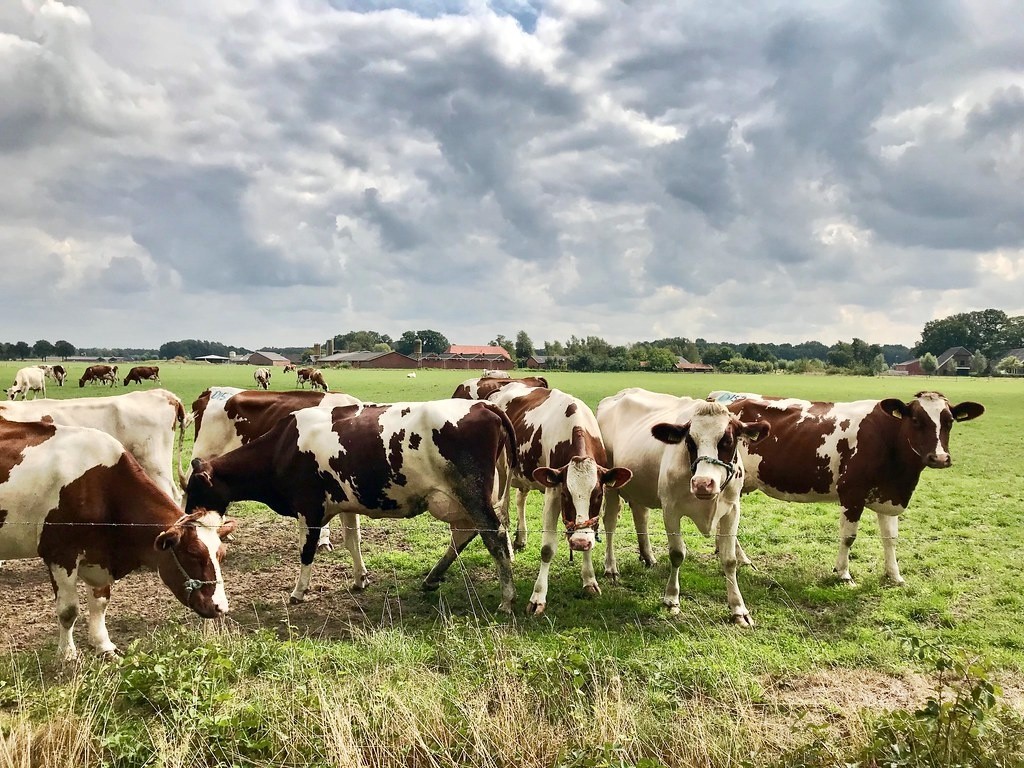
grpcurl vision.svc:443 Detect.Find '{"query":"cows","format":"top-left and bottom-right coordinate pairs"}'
top-left (451, 377), bottom-right (548, 400)
top-left (1, 417), bottom-right (237, 667)
top-left (254, 368), bottom-right (272, 391)
top-left (481, 369), bottom-right (512, 378)
top-left (79, 365), bottom-right (121, 388)
top-left (0, 388), bottom-right (187, 509)
top-left (596, 388), bottom-right (772, 631)
top-left (177, 385), bottom-right (520, 621)
top-left (407, 370), bottom-right (416, 378)
top-left (297, 367), bottom-right (330, 392)
top-left (484, 381), bottom-right (633, 619)
top-left (705, 390), bottom-right (986, 588)
top-left (283, 365), bottom-right (297, 373)
top-left (3, 367), bottom-right (47, 401)
top-left (123, 366), bottom-right (162, 387)
top-left (35, 364), bottom-right (67, 386)
